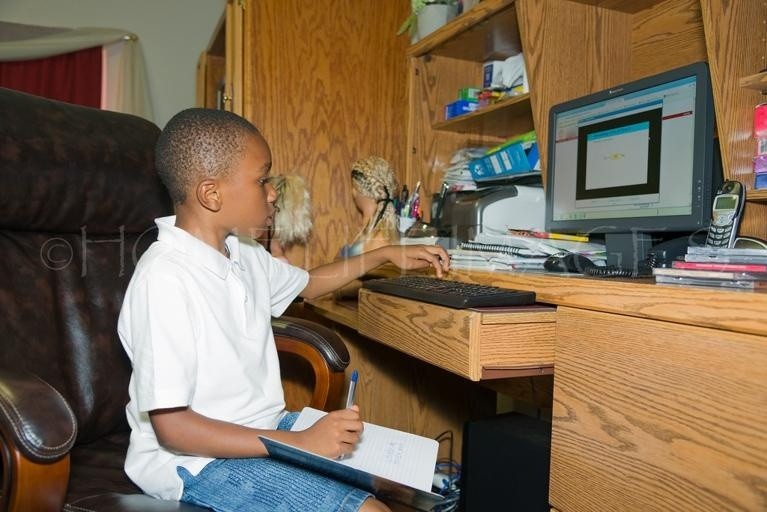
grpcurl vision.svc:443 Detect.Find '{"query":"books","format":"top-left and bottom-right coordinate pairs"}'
top-left (259, 406), bottom-right (445, 512)
top-left (446, 234), bottom-right (556, 271)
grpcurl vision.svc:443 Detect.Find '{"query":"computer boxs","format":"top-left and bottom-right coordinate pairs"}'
top-left (459, 410), bottom-right (550, 512)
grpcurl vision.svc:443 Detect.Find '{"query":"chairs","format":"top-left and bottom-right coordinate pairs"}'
top-left (1, 88), bottom-right (351, 512)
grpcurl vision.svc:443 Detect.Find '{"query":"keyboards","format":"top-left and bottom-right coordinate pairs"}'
top-left (364, 275), bottom-right (537, 310)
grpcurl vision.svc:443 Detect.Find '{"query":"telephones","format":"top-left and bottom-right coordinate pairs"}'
top-left (639, 236), bottom-right (689, 275)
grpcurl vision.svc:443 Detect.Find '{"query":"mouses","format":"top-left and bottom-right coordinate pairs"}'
top-left (545, 252), bottom-right (597, 274)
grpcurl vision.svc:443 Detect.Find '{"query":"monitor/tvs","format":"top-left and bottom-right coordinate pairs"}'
top-left (544, 60), bottom-right (715, 278)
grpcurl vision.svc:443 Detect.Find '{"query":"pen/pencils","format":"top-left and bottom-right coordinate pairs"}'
top-left (401, 181), bottom-right (422, 217)
top-left (430, 183), bottom-right (449, 226)
top-left (340, 370), bottom-right (359, 461)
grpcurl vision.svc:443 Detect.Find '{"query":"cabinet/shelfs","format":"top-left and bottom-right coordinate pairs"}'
top-left (700, 1), bottom-right (767, 200)
top-left (411, 1), bottom-right (531, 130)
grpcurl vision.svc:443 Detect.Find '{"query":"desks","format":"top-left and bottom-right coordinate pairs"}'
top-left (305, 269), bottom-right (766, 512)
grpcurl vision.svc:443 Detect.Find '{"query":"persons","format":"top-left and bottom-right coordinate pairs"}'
top-left (118, 108), bottom-right (449, 510)
top-left (259, 174), bottom-right (312, 264)
top-left (335, 156), bottom-right (398, 261)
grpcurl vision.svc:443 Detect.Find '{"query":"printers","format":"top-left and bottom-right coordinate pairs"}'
top-left (433, 184), bottom-right (545, 251)
